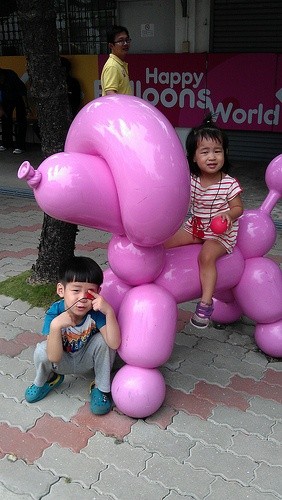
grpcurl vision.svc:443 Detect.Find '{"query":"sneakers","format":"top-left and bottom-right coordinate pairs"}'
top-left (190, 298), bottom-right (215, 330)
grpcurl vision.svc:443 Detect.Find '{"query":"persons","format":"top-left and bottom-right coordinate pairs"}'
top-left (0, 69), bottom-right (27, 153)
top-left (61, 57), bottom-right (82, 115)
top-left (25, 256), bottom-right (121, 414)
top-left (164, 114), bottom-right (243, 329)
top-left (101, 25), bottom-right (132, 96)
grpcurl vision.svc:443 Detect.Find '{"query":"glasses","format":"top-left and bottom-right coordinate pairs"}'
top-left (113, 38), bottom-right (132, 45)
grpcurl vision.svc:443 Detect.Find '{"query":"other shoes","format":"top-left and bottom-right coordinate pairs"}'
top-left (0, 146), bottom-right (8, 151)
top-left (13, 149), bottom-right (23, 155)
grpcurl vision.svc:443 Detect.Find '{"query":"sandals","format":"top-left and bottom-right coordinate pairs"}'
top-left (24, 371), bottom-right (64, 403)
top-left (89, 380), bottom-right (111, 416)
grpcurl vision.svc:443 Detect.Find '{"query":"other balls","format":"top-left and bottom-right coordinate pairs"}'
top-left (210, 217), bottom-right (227, 234)
